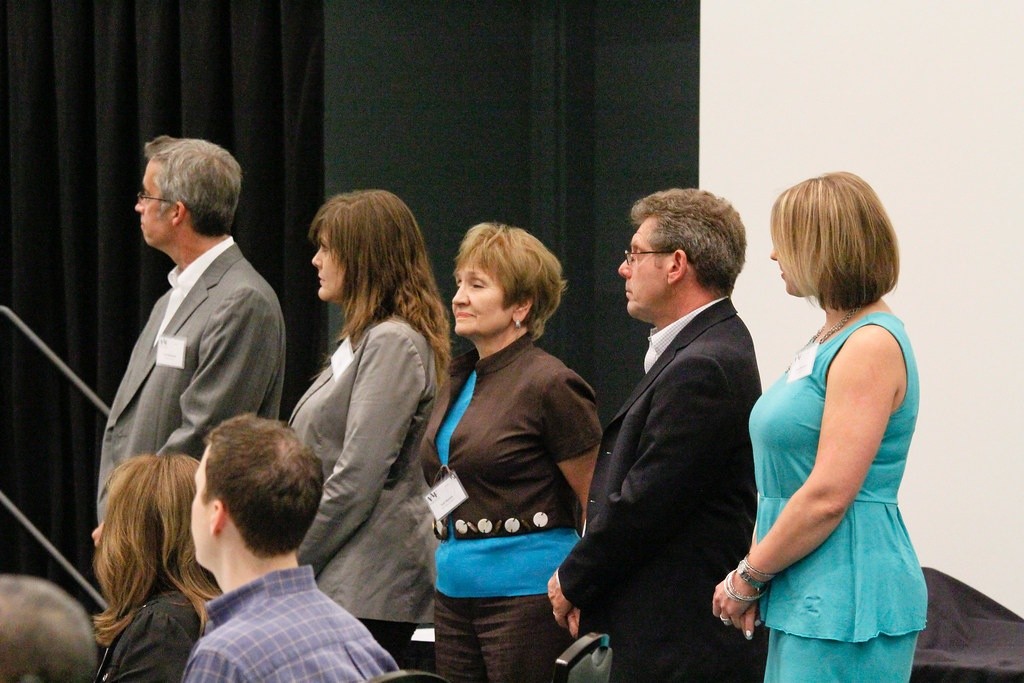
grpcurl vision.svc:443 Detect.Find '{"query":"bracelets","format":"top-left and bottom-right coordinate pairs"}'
top-left (736, 560), bottom-right (765, 589)
top-left (724, 569), bottom-right (768, 602)
top-left (744, 555), bottom-right (776, 578)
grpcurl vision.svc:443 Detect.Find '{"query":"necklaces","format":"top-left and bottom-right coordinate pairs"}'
top-left (784, 306), bottom-right (860, 373)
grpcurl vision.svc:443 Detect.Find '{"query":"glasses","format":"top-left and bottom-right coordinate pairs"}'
top-left (624, 249), bottom-right (695, 267)
top-left (137, 191), bottom-right (171, 204)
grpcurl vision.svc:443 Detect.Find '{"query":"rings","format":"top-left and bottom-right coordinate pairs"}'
top-left (719, 616), bottom-right (732, 625)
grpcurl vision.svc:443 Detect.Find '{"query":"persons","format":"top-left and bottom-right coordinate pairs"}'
top-left (181, 413), bottom-right (399, 683)
top-left (420, 222), bottom-right (602, 683)
top-left (92, 453), bottom-right (223, 683)
top-left (90, 136), bottom-right (287, 549)
top-left (0, 574), bottom-right (97, 683)
top-left (713, 171), bottom-right (928, 683)
top-left (288, 189), bottom-right (451, 670)
top-left (548, 189), bottom-right (762, 683)
